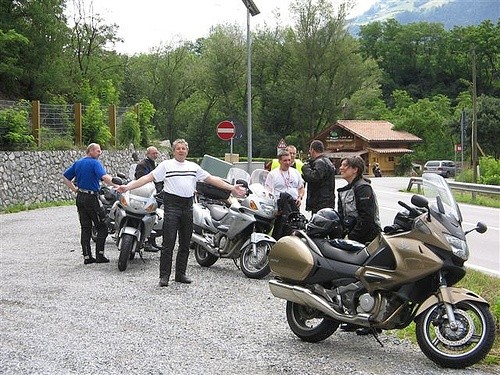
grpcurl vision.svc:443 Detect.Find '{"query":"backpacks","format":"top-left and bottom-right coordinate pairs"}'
top-left (272, 192), bottom-right (300, 241)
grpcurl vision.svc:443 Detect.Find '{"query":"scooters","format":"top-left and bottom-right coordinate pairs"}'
top-left (369, 162), bottom-right (382, 177)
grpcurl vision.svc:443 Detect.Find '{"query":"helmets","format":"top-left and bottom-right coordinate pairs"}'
top-left (306, 208), bottom-right (343, 239)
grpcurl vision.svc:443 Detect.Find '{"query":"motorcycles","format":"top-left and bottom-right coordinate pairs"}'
top-left (267, 173), bottom-right (496, 369)
top-left (90, 152), bottom-right (142, 242)
top-left (109, 176), bottom-right (165, 272)
top-left (187, 167), bottom-right (279, 279)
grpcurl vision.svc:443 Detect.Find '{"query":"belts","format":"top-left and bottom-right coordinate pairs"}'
top-left (79, 189), bottom-right (95, 194)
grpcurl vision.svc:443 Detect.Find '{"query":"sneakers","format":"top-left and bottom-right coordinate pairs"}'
top-left (148, 238), bottom-right (163, 250)
top-left (84, 255), bottom-right (96, 264)
top-left (345, 323), bottom-right (363, 331)
top-left (356, 327), bottom-right (382, 335)
top-left (175, 274), bottom-right (191, 283)
top-left (144, 241), bottom-right (159, 252)
top-left (160, 275), bottom-right (169, 286)
top-left (96, 252), bottom-right (109, 263)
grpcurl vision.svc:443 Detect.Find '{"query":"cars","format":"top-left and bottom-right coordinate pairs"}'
top-left (423, 160), bottom-right (458, 178)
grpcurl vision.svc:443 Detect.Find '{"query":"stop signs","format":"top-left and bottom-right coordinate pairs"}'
top-left (216, 121), bottom-right (236, 141)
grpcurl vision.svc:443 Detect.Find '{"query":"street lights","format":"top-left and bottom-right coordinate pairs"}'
top-left (242, 0), bottom-right (262, 174)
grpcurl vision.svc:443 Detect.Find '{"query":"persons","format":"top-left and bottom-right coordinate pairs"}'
top-left (135, 147), bottom-right (163, 193)
top-left (63, 143), bottom-right (112, 264)
top-left (337, 155), bottom-right (383, 245)
top-left (117, 139), bottom-right (246, 287)
top-left (271, 145), bottom-right (304, 169)
top-left (266, 152), bottom-right (305, 207)
top-left (301, 139), bottom-right (336, 214)
